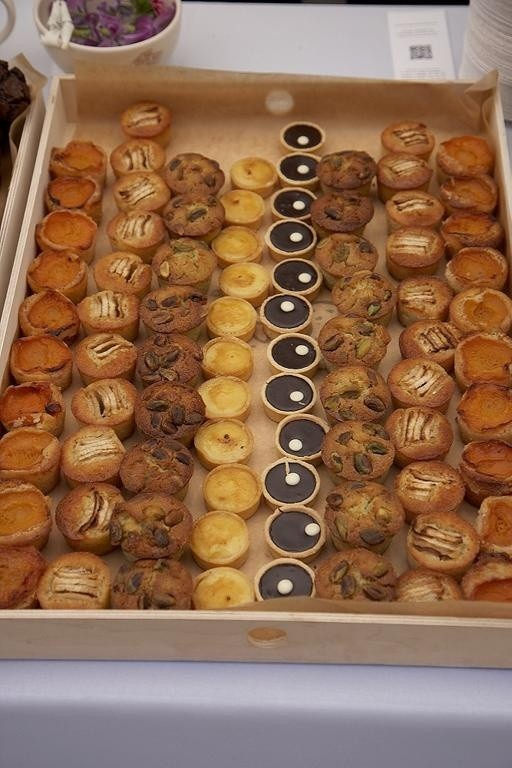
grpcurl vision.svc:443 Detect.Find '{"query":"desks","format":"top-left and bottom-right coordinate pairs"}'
top-left (1, 0), bottom-right (512, 768)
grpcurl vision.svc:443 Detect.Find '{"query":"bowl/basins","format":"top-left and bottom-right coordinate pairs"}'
top-left (33, 0), bottom-right (183, 78)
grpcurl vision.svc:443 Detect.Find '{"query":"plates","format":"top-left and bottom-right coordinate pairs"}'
top-left (459, 0), bottom-right (511, 123)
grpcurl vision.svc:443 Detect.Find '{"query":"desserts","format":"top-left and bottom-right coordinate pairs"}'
top-left (0, 100), bottom-right (511, 610)
